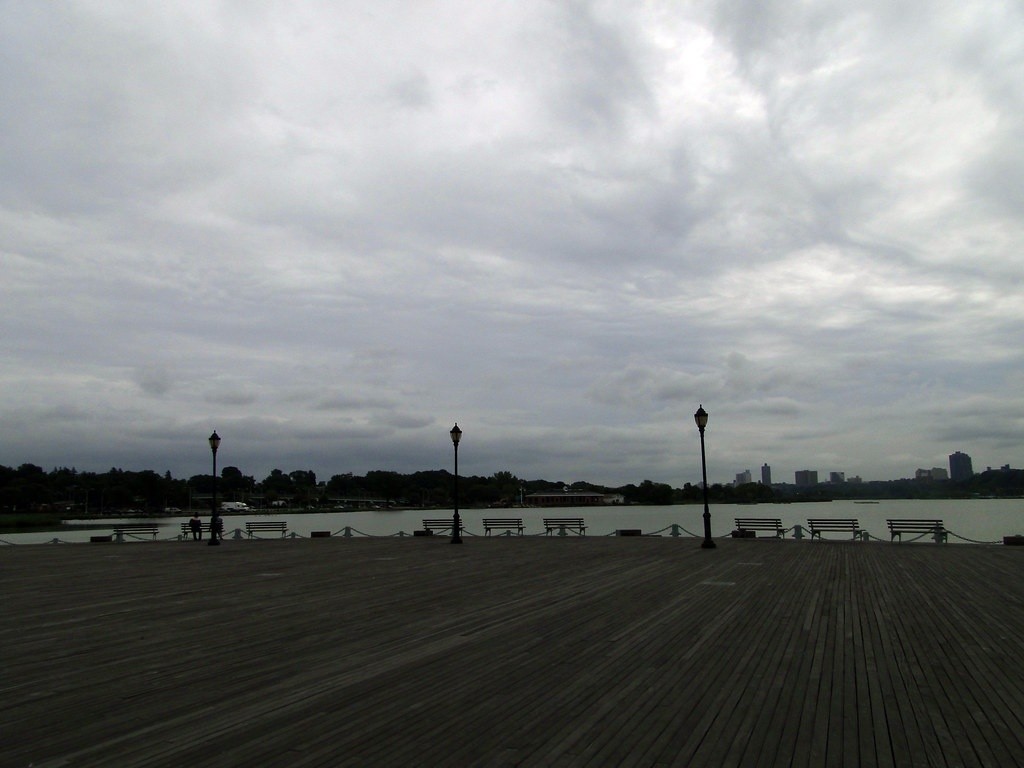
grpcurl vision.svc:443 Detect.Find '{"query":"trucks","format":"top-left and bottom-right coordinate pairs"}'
top-left (221, 501), bottom-right (249, 513)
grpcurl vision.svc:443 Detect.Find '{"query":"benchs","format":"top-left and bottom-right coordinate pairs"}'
top-left (113, 524), bottom-right (159, 542)
top-left (246, 522), bottom-right (289, 539)
top-left (886, 519), bottom-right (951, 545)
top-left (543, 518), bottom-right (588, 536)
top-left (735, 518), bottom-right (788, 539)
top-left (482, 519), bottom-right (526, 536)
top-left (422, 519), bottom-right (465, 537)
top-left (807, 519), bottom-right (866, 542)
top-left (181, 522), bottom-right (225, 540)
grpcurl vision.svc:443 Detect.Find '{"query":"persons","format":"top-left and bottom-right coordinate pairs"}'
top-left (189, 512), bottom-right (202, 541)
top-left (210, 513), bottom-right (224, 541)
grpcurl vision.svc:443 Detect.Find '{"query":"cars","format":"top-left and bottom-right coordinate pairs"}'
top-left (164, 508), bottom-right (182, 513)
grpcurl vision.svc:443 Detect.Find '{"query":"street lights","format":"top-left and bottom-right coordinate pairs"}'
top-left (694, 404), bottom-right (716, 549)
top-left (207, 430), bottom-right (221, 547)
top-left (449, 423), bottom-right (463, 545)
